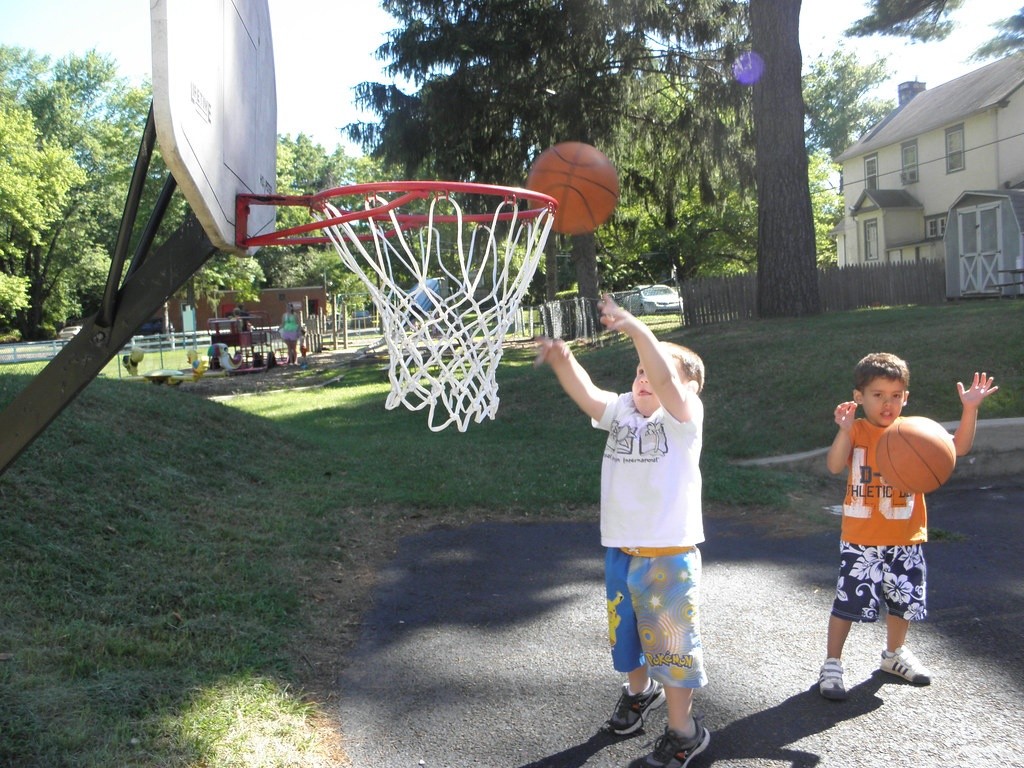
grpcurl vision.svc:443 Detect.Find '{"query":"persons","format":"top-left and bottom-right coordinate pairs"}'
top-left (535, 296), bottom-right (711, 768)
top-left (820, 352), bottom-right (998, 699)
top-left (278, 304), bottom-right (301, 364)
top-left (232, 303), bottom-right (255, 332)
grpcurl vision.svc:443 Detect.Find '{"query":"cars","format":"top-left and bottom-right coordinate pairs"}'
top-left (58, 326), bottom-right (82, 337)
top-left (618, 284), bottom-right (685, 315)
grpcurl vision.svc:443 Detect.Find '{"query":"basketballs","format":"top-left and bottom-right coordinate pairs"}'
top-left (875, 414), bottom-right (959, 499)
top-left (526, 139), bottom-right (621, 234)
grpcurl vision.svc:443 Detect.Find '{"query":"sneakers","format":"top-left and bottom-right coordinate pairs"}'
top-left (881, 646), bottom-right (931, 685)
top-left (646, 715), bottom-right (710, 768)
top-left (820, 661), bottom-right (846, 700)
top-left (611, 677), bottom-right (666, 734)
